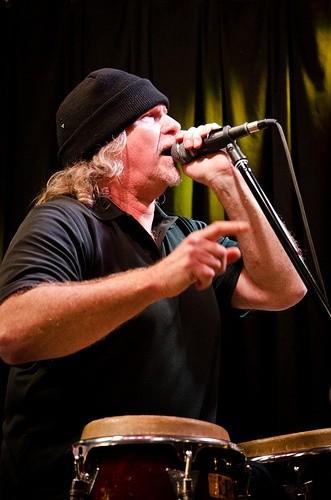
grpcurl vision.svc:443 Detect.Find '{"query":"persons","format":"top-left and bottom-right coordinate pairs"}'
top-left (0, 69), bottom-right (308, 500)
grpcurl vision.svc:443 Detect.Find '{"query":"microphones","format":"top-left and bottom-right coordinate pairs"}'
top-left (170, 119), bottom-right (277, 165)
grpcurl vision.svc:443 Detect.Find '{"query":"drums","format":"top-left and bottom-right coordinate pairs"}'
top-left (238, 429), bottom-right (331, 499)
top-left (68, 415), bottom-right (249, 500)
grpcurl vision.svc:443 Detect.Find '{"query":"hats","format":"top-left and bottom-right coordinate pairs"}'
top-left (57, 68), bottom-right (169, 169)
